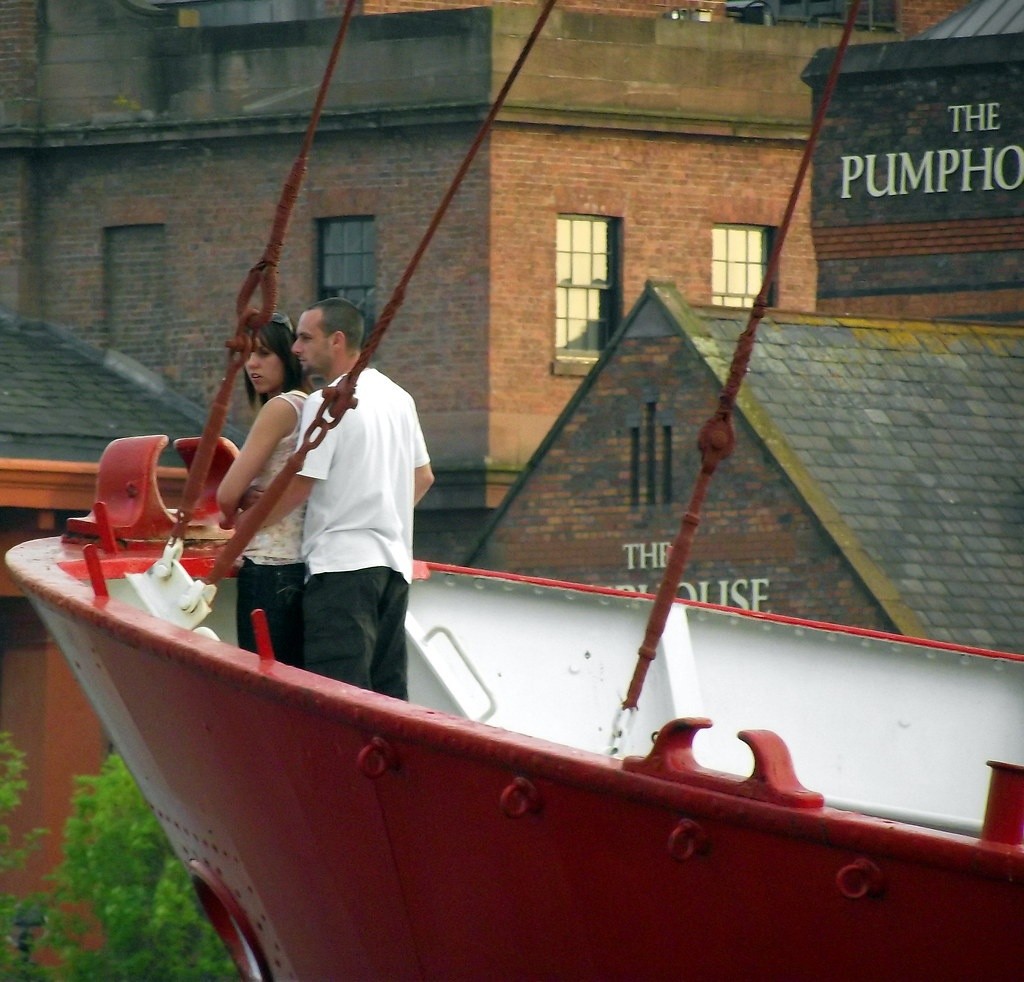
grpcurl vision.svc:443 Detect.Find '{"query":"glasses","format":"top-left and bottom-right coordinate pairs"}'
top-left (250, 307), bottom-right (294, 337)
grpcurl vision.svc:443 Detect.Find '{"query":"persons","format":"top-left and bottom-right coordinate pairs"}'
top-left (236, 297), bottom-right (435, 702)
top-left (216, 311), bottom-right (310, 672)
